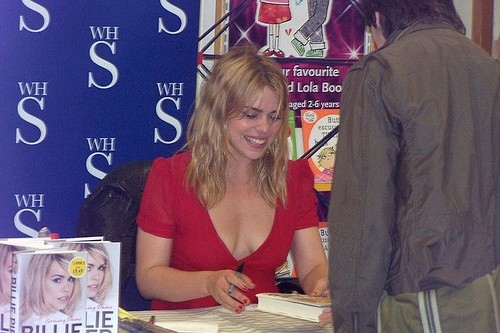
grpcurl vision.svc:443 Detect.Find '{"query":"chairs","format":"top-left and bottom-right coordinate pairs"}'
top-left (77, 161), bottom-right (153, 312)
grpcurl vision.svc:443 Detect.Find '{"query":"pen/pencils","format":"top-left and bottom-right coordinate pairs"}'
top-left (149, 315), bottom-right (155, 324)
top-left (228, 261), bottom-right (245, 295)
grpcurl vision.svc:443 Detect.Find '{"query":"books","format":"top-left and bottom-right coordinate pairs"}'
top-left (300, 108), bottom-right (339, 191)
top-left (288, 222), bottom-right (330, 278)
top-left (254, 293), bottom-right (331, 322)
top-left (288, 108), bottom-right (298, 161)
top-left (363, 25), bottom-right (377, 55)
top-left (0, 236), bottom-right (122, 333)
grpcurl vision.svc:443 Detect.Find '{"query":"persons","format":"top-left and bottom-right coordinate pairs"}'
top-left (26, 254), bottom-right (82, 325)
top-left (134, 45), bottom-right (329, 313)
top-left (0, 242), bottom-right (28, 312)
top-left (317, 0), bottom-right (500, 333)
top-left (59, 243), bottom-right (113, 310)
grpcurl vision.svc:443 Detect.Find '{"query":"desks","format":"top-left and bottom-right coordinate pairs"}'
top-left (119, 305), bottom-right (334, 333)
